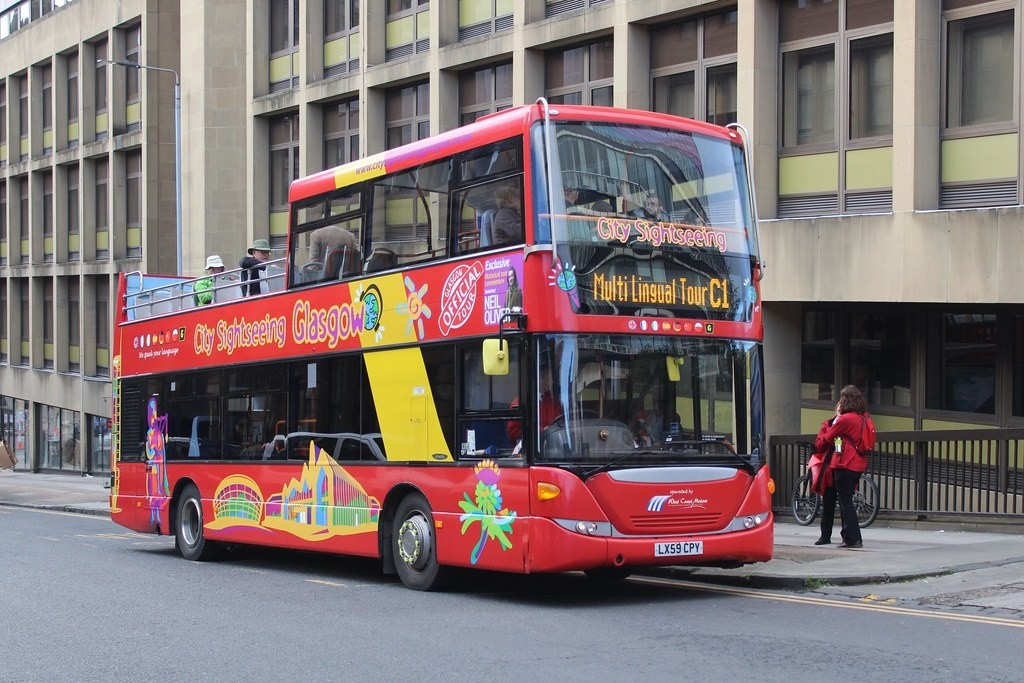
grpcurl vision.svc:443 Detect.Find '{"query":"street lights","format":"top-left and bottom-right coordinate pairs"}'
top-left (115, 61), bottom-right (184, 277)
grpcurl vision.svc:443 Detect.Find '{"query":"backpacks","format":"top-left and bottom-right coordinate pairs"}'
top-left (847, 411), bottom-right (875, 455)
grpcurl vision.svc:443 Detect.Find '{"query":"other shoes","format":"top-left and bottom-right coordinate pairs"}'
top-left (815, 538), bottom-right (831, 545)
top-left (845, 538), bottom-right (861, 546)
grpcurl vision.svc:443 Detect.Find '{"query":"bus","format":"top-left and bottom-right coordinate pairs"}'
top-left (107, 96), bottom-right (777, 590)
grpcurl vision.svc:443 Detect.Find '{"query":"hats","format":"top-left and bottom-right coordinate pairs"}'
top-left (247, 239), bottom-right (272, 255)
top-left (205, 254), bottom-right (224, 269)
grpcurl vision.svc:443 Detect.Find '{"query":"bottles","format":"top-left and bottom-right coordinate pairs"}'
top-left (835, 437), bottom-right (842, 453)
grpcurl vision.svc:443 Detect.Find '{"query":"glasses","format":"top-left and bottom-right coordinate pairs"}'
top-left (645, 201), bottom-right (659, 207)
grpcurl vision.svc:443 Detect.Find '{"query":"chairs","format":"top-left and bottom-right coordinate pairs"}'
top-left (479, 209), bottom-right (495, 248)
top-left (133, 245), bottom-right (398, 319)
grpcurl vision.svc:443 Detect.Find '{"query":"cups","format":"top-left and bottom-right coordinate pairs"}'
top-left (484, 445), bottom-right (498, 455)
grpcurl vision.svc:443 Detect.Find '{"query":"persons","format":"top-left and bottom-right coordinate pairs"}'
top-left (681, 208), bottom-right (706, 227)
top-left (562, 183), bottom-right (579, 208)
top-left (633, 193), bottom-right (663, 222)
top-left (490, 187), bottom-right (523, 245)
top-left (811, 386), bottom-right (876, 549)
top-left (194, 255), bottom-right (238, 307)
top-left (239, 239), bottom-right (272, 298)
top-left (309, 211), bottom-right (357, 271)
top-left (507, 364), bottom-right (565, 451)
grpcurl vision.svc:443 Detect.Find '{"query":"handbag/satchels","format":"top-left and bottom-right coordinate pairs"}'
top-left (807, 453), bottom-right (828, 496)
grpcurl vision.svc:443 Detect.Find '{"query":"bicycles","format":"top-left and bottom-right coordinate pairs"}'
top-left (791, 440), bottom-right (881, 528)
